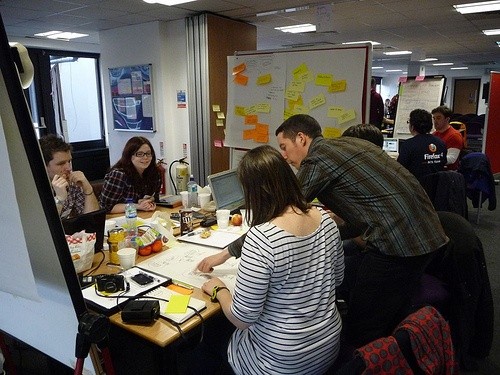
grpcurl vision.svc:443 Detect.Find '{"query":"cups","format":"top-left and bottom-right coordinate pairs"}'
top-left (216, 209), bottom-right (233, 228)
top-left (180, 191), bottom-right (188, 205)
top-left (117, 248), bottom-right (137, 270)
top-left (198, 193), bottom-right (211, 208)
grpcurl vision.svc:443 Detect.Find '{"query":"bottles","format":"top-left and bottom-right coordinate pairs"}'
top-left (187, 173), bottom-right (199, 208)
top-left (106, 227), bottom-right (126, 266)
top-left (126, 198), bottom-right (139, 235)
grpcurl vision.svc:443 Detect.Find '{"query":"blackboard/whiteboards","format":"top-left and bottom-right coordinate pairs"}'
top-left (2, 31), bottom-right (103, 374)
top-left (393, 75), bottom-right (447, 139)
top-left (223, 41), bottom-right (374, 150)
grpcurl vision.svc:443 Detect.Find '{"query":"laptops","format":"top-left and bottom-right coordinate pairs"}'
top-left (207, 169), bottom-right (249, 220)
top-left (383, 138), bottom-right (399, 159)
top-left (62, 208), bottom-right (107, 252)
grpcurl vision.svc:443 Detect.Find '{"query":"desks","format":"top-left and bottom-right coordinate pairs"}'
top-left (64, 205), bottom-right (254, 375)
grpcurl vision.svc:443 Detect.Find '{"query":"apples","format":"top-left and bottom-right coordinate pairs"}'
top-left (231, 214), bottom-right (242, 225)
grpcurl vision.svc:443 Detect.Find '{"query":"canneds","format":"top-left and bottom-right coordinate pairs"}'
top-left (108, 228), bottom-right (125, 264)
top-left (179, 208), bottom-right (193, 236)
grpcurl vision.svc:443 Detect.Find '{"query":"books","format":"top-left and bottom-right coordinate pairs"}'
top-left (137, 285), bottom-right (207, 325)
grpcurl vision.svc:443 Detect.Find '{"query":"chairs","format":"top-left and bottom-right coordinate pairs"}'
top-left (454, 152), bottom-right (496, 223)
top-left (417, 171), bottom-right (468, 223)
top-left (324, 298), bottom-right (458, 374)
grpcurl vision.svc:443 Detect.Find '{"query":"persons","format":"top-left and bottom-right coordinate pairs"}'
top-left (370, 78), bottom-right (385, 130)
top-left (98, 136), bottom-right (162, 214)
top-left (396, 109), bottom-right (447, 180)
top-left (196, 114), bottom-right (450, 375)
top-left (342, 95), bottom-right (400, 149)
top-left (431, 106), bottom-right (464, 171)
top-left (37, 133), bottom-right (100, 221)
top-left (201, 144), bottom-right (345, 375)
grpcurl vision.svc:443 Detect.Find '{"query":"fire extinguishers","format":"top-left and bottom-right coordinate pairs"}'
top-left (157, 159), bottom-right (167, 194)
top-left (168, 157), bottom-right (189, 195)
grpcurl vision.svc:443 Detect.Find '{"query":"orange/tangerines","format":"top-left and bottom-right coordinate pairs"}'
top-left (151, 240), bottom-right (162, 252)
top-left (139, 247), bottom-right (151, 255)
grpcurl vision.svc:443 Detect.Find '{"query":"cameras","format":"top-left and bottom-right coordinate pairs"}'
top-left (97, 274), bottom-right (125, 292)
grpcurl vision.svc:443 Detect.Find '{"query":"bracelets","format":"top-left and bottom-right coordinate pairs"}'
top-left (83, 191), bottom-right (94, 195)
top-left (211, 286), bottom-right (230, 300)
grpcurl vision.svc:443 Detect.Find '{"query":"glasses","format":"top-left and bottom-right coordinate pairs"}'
top-left (407, 120), bottom-right (412, 124)
top-left (134, 151), bottom-right (153, 158)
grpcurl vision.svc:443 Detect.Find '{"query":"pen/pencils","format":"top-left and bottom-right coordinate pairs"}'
top-left (149, 183), bottom-right (160, 199)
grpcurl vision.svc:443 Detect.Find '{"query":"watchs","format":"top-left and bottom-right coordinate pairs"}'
top-left (53, 196), bottom-right (68, 206)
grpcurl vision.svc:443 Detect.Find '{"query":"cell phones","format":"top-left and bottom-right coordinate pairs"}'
top-left (131, 273), bottom-right (153, 285)
